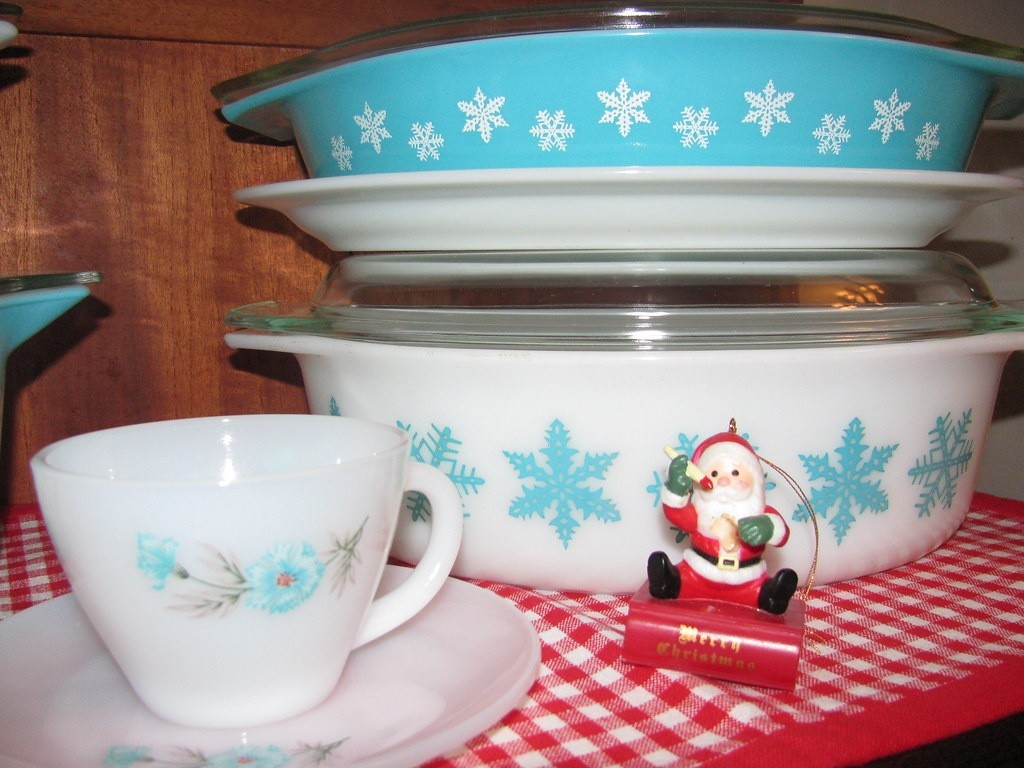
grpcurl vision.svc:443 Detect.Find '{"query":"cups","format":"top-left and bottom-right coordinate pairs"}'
top-left (30, 413), bottom-right (465, 729)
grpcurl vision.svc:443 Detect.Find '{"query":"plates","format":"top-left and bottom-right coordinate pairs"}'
top-left (0, 561), bottom-right (544, 768)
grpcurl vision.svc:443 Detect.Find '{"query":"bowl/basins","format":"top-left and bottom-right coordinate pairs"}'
top-left (204, 2), bottom-right (1024, 599)
